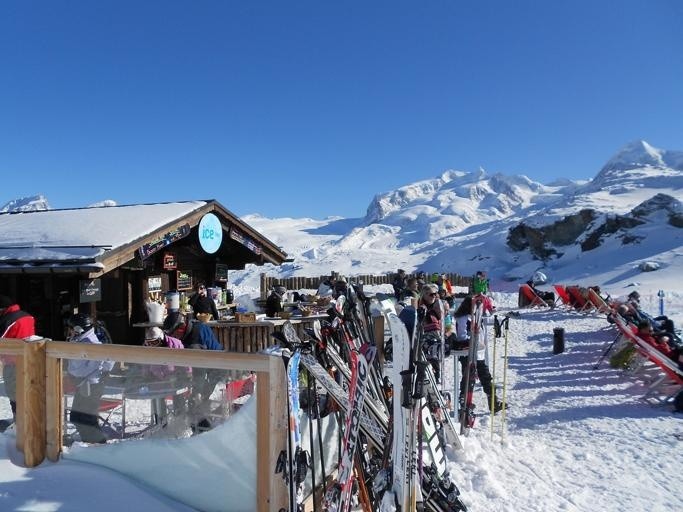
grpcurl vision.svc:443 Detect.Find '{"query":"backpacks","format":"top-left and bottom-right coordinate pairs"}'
top-left (398, 301), bottom-right (425, 343)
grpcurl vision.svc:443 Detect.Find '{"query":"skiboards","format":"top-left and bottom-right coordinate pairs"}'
top-left (275, 348), bottom-right (313, 512)
top-left (460, 408), bottom-right (470, 438)
top-left (474, 404), bottom-right (513, 418)
top-left (419, 348), bottom-right (464, 450)
top-left (304, 278), bottom-right (446, 512)
top-left (388, 310), bottom-right (416, 512)
top-left (283, 322), bottom-right (469, 511)
top-left (260, 273), bottom-right (484, 512)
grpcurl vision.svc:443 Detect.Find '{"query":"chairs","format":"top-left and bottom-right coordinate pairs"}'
top-left (518, 284), bottom-right (683, 408)
top-left (63, 375), bottom-right (255, 447)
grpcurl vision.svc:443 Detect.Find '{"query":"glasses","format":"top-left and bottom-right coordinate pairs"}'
top-left (427, 293), bottom-right (436, 297)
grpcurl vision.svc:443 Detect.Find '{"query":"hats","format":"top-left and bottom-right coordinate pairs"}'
top-left (438, 286), bottom-right (446, 294)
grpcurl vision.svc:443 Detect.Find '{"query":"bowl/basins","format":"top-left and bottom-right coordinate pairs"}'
top-left (299, 310), bottom-right (313, 316)
top-left (279, 311), bottom-right (293, 319)
top-left (196, 314), bottom-right (213, 323)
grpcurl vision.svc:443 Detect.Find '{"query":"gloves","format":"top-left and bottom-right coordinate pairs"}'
top-left (399, 360), bottom-right (429, 409)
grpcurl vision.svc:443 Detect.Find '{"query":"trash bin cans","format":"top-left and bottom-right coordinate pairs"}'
top-left (553, 327), bottom-right (564, 355)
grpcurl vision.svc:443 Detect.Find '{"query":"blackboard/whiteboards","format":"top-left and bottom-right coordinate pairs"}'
top-left (215, 264), bottom-right (228, 282)
top-left (176, 270), bottom-right (193, 291)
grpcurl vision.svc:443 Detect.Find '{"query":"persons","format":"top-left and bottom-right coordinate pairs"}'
top-left (162, 292), bottom-right (187, 342)
top-left (184, 313), bottom-right (225, 400)
top-left (392, 269), bottom-right (508, 428)
top-left (266, 284), bottom-right (288, 317)
top-left (144, 325), bottom-right (193, 432)
top-left (587, 282), bottom-right (683, 366)
top-left (188, 283), bottom-right (219, 320)
top-left (527, 280), bottom-right (557, 305)
top-left (61, 315), bottom-right (114, 442)
top-left (0, 288), bottom-right (36, 419)
top-left (314, 276), bottom-right (336, 297)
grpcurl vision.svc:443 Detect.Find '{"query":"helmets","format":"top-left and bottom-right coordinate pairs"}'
top-left (145, 312), bottom-right (192, 347)
top-left (67, 313), bottom-right (91, 336)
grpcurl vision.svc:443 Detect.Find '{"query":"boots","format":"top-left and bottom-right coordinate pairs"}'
top-left (488, 396), bottom-right (509, 413)
top-left (460, 403), bottom-right (476, 428)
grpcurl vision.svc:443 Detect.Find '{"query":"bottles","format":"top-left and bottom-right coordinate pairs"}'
top-left (148, 291), bottom-right (162, 305)
